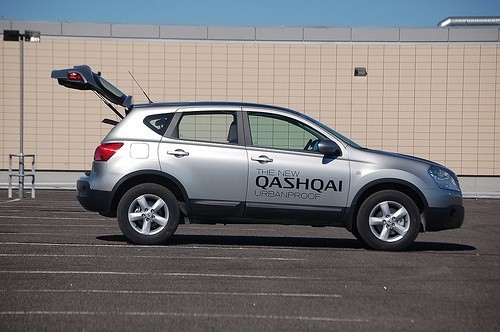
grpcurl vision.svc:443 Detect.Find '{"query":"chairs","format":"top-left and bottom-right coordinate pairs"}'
top-left (227, 121), bottom-right (238, 145)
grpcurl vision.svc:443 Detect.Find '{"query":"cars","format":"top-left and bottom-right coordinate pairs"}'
top-left (51, 64), bottom-right (465, 251)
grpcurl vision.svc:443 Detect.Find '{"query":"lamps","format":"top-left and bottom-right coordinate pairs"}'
top-left (354, 67), bottom-right (367, 76)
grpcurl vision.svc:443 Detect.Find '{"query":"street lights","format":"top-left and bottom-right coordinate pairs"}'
top-left (2, 28), bottom-right (42, 200)
